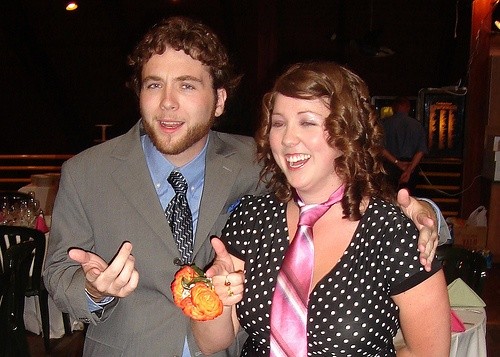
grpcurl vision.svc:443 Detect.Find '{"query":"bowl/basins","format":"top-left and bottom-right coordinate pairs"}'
top-left (32, 175), bottom-right (53, 187)
top-left (45, 173), bottom-right (62, 184)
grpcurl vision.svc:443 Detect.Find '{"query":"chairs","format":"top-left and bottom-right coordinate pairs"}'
top-left (436, 244), bottom-right (486, 298)
top-left (0, 225), bottom-right (71, 357)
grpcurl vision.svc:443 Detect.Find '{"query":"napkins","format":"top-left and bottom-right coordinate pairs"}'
top-left (450, 309), bottom-right (465, 332)
top-left (447, 278), bottom-right (487, 307)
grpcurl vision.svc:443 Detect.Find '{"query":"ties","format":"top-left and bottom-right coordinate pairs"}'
top-left (269, 182), bottom-right (347, 357)
top-left (164, 172), bottom-right (194, 267)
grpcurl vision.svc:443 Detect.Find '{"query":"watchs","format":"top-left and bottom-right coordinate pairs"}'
top-left (395, 159), bottom-right (398, 164)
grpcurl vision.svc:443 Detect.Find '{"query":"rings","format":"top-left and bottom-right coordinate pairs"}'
top-left (225, 275), bottom-right (231, 286)
top-left (227, 286), bottom-right (233, 297)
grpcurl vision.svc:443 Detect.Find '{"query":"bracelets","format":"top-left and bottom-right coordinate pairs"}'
top-left (84, 289), bottom-right (104, 299)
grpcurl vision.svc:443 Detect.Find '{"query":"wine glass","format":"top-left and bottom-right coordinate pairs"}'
top-left (0, 191), bottom-right (40, 229)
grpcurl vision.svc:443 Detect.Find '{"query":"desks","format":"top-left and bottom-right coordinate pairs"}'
top-left (18, 184), bottom-right (58, 215)
top-left (393, 307), bottom-right (488, 357)
top-left (0, 216), bottom-right (84, 339)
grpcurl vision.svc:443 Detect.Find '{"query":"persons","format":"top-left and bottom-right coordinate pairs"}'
top-left (190, 62), bottom-right (450, 357)
top-left (43, 17), bottom-right (451, 357)
top-left (378, 94), bottom-right (427, 197)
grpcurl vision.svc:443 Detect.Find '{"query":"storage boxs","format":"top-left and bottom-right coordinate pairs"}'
top-left (451, 217), bottom-right (487, 252)
